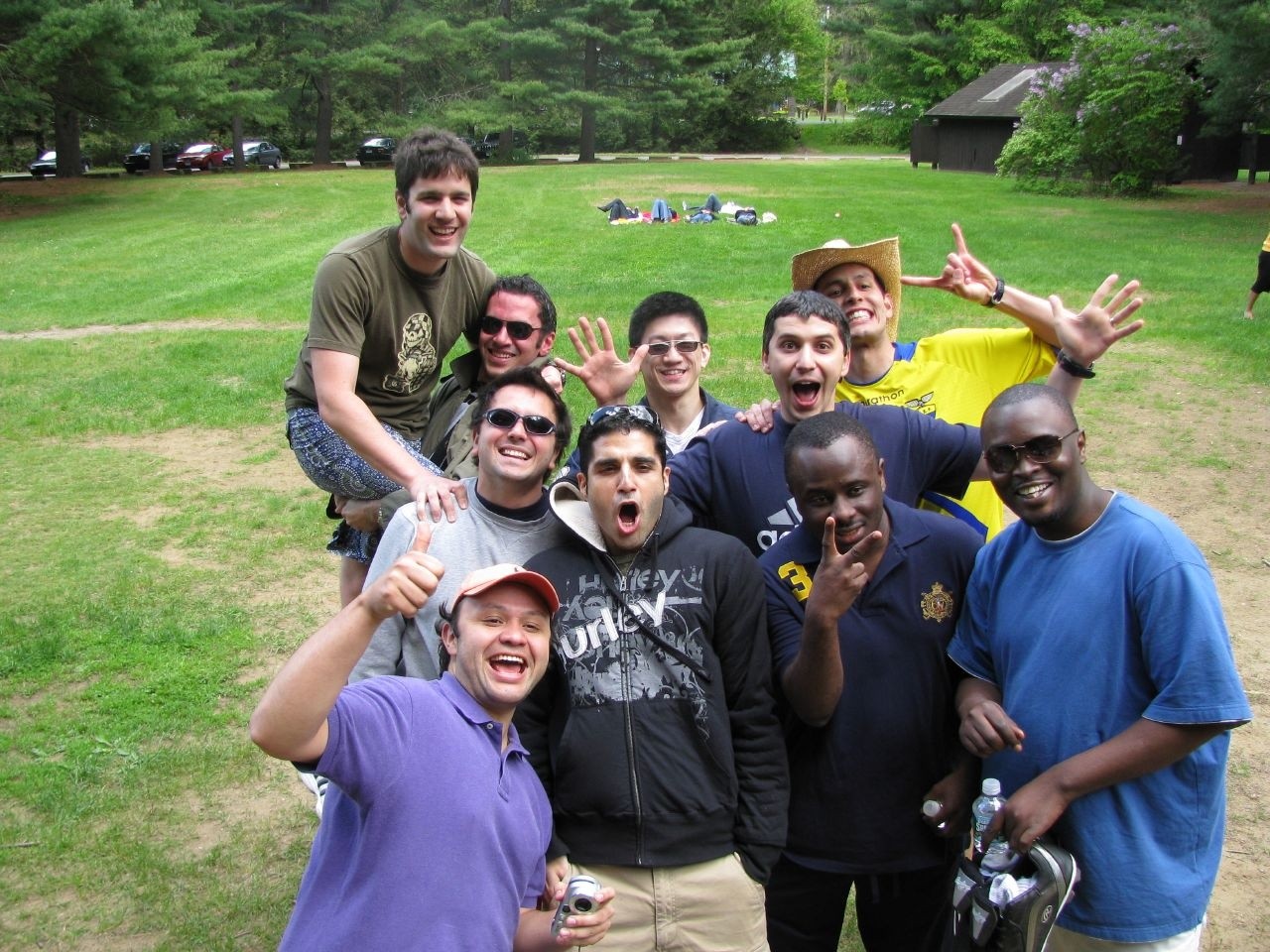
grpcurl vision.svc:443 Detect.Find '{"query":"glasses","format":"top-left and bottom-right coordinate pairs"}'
top-left (981, 429), bottom-right (1079, 474)
top-left (587, 405), bottom-right (662, 431)
top-left (481, 315), bottom-right (545, 340)
top-left (482, 408), bottom-right (558, 436)
top-left (636, 341), bottom-right (704, 356)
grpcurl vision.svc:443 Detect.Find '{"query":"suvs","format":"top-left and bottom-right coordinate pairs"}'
top-left (455, 131), bottom-right (529, 160)
top-left (122, 142), bottom-right (185, 173)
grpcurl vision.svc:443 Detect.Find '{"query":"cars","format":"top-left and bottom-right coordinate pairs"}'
top-left (222, 141), bottom-right (283, 169)
top-left (356, 137), bottom-right (399, 166)
top-left (176, 142), bottom-right (233, 170)
top-left (29, 151), bottom-right (91, 178)
top-left (856, 100), bottom-right (896, 116)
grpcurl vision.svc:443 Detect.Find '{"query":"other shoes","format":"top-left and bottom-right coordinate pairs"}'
top-left (298, 770), bottom-right (329, 821)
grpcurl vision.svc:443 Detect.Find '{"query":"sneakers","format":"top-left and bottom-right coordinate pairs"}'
top-left (942, 852), bottom-right (991, 952)
top-left (969, 838), bottom-right (1081, 952)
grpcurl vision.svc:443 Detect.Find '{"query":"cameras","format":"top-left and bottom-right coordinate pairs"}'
top-left (550, 875), bottom-right (601, 936)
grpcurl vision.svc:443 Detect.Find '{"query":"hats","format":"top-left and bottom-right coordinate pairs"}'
top-left (451, 563), bottom-right (560, 619)
top-left (792, 236), bottom-right (902, 342)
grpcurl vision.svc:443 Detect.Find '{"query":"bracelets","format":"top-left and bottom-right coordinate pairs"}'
top-left (377, 508), bottom-right (382, 526)
top-left (985, 277), bottom-right (1004, 308)
top-left (1057, 350), bottom-right (1096, 379)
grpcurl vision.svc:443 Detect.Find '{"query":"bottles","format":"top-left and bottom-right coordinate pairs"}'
top-left (971, 778), bottom-right (1009, 855)
top-left (923, 800), bottom-right (945, 829)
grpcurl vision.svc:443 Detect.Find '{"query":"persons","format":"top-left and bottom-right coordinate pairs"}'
top-left (284, 128), bottom-right (497, 611)
top-left (792, 223), bottom-right (1063, 544)
top-left (554, 290), bottom-right (781, 484)
top-left (945, 383), bottom-right (1253, 952)
top-left (511, 404), bottom-right (792, 952)
top-left (598, 198), bottom-right (639, 222)
top-left (251, 520), bottom-right (617, 952)
top-left (735, 209), bottom-right (756, 225)
top-left (1244, 232), bottom-right (1270, 319)
top-left (756, 411), bottom-right (984, 952)
top-left (682, 194), bottom-right (743, 214)
top-left (650, 199), bottom-right (677, 223)
top-left (554, 274), bottom-right (1146, 557)
top-left (334, 275), bottom-right (558, 532)
top-left (350, 366), bottom-right (572, 686)
top-left (685, 210), bottom-right (715, 223)
